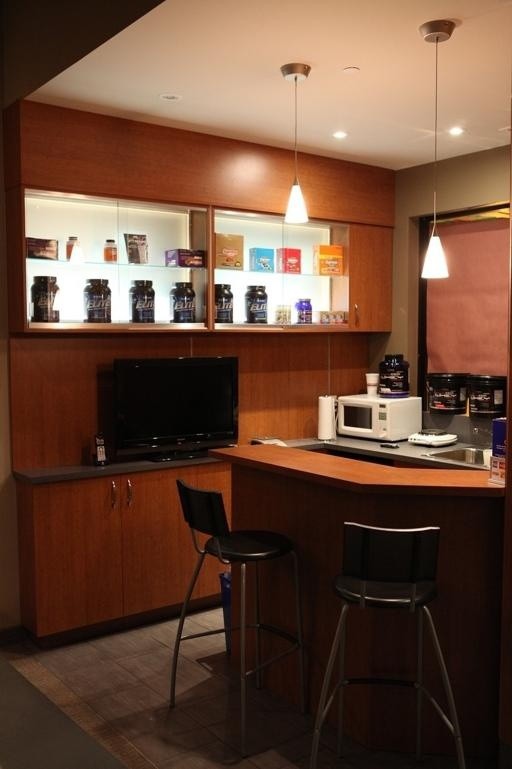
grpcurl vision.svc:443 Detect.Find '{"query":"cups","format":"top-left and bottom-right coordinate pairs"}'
top-left (365, 373), bottom-right (379, 395)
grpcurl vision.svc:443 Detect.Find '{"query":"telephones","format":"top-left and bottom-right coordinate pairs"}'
top-left (92, 432), bottom-right (110, 466)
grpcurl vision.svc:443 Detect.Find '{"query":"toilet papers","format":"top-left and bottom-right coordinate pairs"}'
top-left (317, 397), bottom-right (337, 441)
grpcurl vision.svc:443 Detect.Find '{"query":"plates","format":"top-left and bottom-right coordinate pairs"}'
top-left (407, 430), bottom-right (458, 447)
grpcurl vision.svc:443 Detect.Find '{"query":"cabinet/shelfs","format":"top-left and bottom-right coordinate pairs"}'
top-left (8, 184), bottom-right (394, 336)
top-left (11, 458), bottom-right (234, 649)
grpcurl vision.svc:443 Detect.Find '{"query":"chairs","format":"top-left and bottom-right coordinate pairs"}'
top-left (170, 479), bottom-right (307, 757)
top-left (310, 522), bottom-right (466, 769)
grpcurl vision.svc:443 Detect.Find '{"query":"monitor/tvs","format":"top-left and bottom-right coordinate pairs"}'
top-left (114, 357), bottom-right (238, 462)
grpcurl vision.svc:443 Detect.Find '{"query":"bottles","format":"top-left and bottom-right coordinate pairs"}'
top-left (274, 298), bottom-right (346, 325)
top-left (30, 274), bottom-right (61, 324)
top-left (425, 372), bottom-right (471, 414)
top-left (214, 284), bottom-right (233, 323)
top-left (468, 374), bottom-right (507, 419)
top-left (84, 277), bottom-right (111, 324)
top-left (378, 353), bottom-right (409, 397)
top-left (170, 281), bottom-right (196, 322)
top-left (129, 280), bottom-right (155, 323)
top-left (244, 285), bottom-right (269, 324)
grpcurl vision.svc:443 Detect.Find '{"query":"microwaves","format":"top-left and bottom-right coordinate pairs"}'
top-left (337, 395), bottom-right (423, 444)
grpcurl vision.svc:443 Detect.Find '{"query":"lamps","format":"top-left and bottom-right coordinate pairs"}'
top-left (281, 63), bottom-right (312, 225)
top-left (418, 20), bottom-right (456, 279)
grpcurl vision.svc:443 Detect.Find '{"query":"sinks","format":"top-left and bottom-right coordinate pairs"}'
top-left (420, 445), bottom-right (493, 467)
